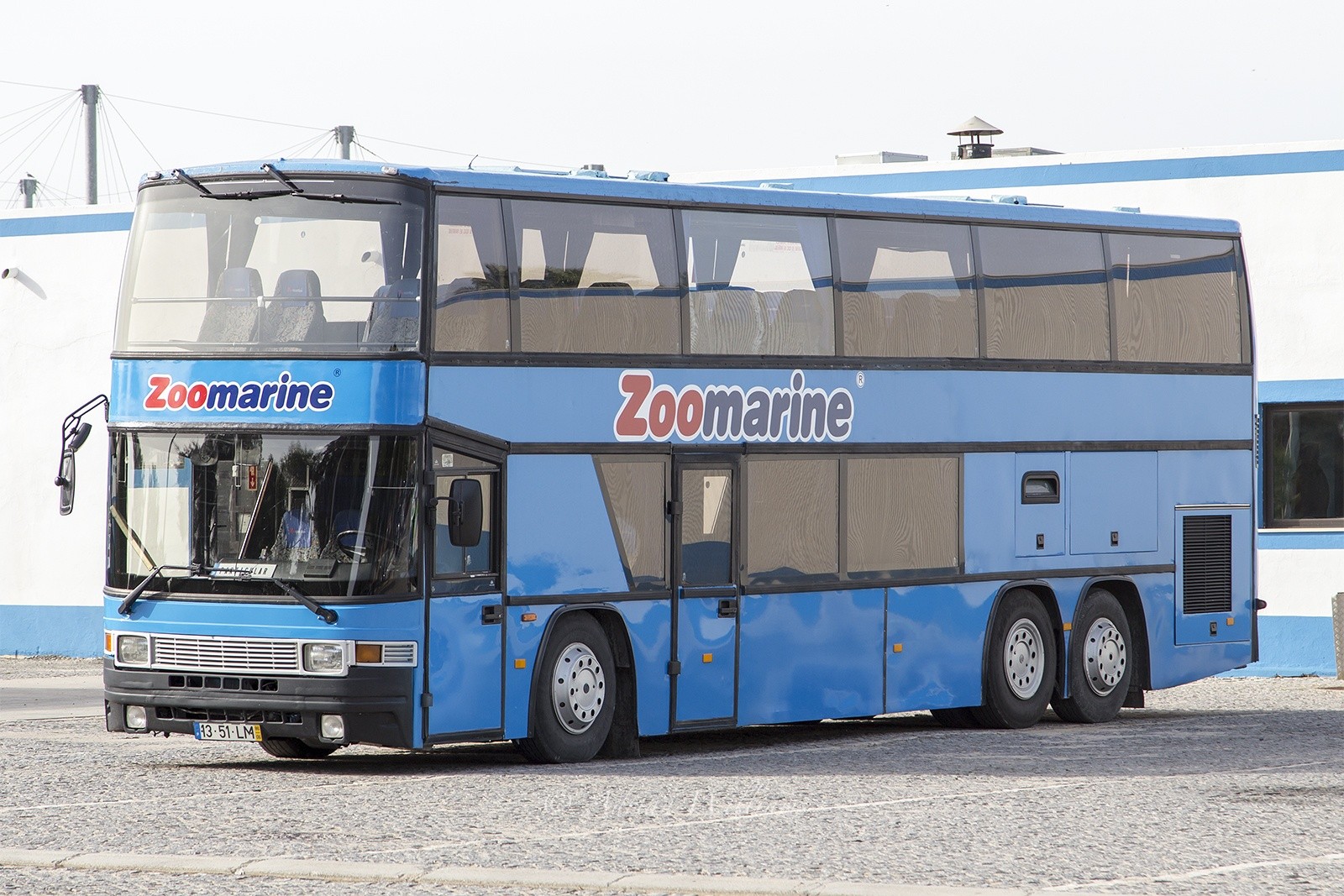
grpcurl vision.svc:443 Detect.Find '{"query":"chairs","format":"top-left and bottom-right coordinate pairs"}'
top-left (194, 257), bottom-right (1097, 359)
top-left (329, 501), bottom-right (373, 572)
top-left (272, 495), bottom-right (320, 562)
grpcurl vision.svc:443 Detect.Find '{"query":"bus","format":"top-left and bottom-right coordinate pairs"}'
top-left (53, 155), bottom-right (1268, 768)
top-left (1211, 379), bottom-right (1343, 680)
